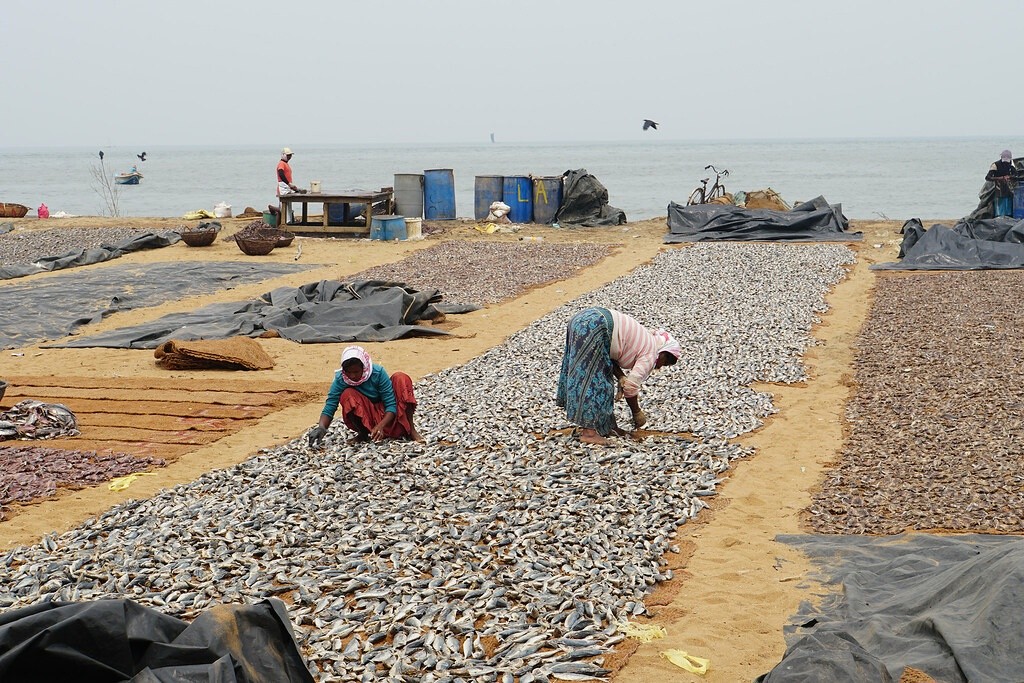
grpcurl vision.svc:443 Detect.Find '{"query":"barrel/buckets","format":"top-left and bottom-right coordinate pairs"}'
top-left (310, 181), bottom-right (321, 194)
top-left (394, 173), bottom-right (425, 217)
top-left (474, 175), bottom-right (503, 220)
top-left (533, 176), bottom-right (563, 224)
top-left (503, 175), bottom-right (532, 224)
top-left (370, 215), bottom-right (406, 241)
top-left (1013, 185), bottom-right (1024, 220)
top-left (423, 169), bottom-right (456, 220)
top-left (405, 217), bottom-right (422, 239)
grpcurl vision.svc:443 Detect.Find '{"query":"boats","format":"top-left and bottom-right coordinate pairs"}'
top-left (115, 165), bottom-right (144, 184)
top-left (0, 202), bottom-right (32, 217)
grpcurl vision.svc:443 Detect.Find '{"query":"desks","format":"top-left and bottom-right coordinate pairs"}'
top-left (276, 190), bottom-right (395, 238)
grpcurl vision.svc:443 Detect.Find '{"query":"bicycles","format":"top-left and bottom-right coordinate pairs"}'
top-left (687, 165), bottom-right (729, 207)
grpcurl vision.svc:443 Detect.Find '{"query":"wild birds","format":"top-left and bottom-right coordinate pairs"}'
top-left (643, 119), bottom-right (659, 130)
top-left (99, 151), bottom-right (104, 159)
top-left (137, 152), bottom-right (146, 161)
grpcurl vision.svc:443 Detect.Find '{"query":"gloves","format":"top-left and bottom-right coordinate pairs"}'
top-left (615, 376), bottom-right (627, 403)
top-left (632, 410), bottom-right (647, 429)
top-left (308, 427), bottom-right (327, 447)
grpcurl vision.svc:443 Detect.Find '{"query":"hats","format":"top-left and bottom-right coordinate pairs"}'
top-left (280, 147), bottom-right (294, 155)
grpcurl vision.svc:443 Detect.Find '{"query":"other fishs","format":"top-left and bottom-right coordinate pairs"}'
top-left (0, 223), bottom-right (1024, 682)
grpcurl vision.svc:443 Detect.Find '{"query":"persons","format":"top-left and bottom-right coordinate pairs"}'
top-left (984, 150), bottom-right (1018, 217)
top-left (130, 164), bottom-right (137, 173)
top-left (307, 345), bottom-right (425, 448)
top-left (275, 146), bottom-right (297, 225)
top-left (556, 306), bottom-right (680, 446)
